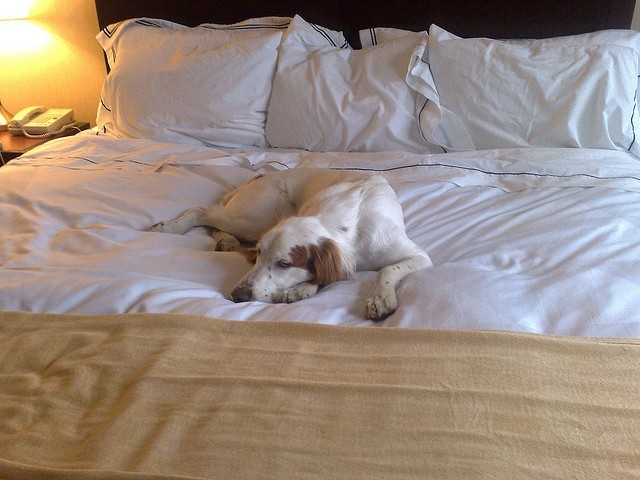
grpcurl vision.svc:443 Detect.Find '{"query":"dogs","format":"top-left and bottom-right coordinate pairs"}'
top-left (143, 165), bottom-right (433, 324)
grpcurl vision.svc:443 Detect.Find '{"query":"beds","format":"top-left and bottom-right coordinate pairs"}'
top-left (0, 1), bottom-right (640, 480)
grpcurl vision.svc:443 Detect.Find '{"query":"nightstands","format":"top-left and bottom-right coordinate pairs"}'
top-left (0, 120), bottom-right (90, 168)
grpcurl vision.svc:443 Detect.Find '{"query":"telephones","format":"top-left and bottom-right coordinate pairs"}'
top-left (8, 106), bottom-right (78, 138)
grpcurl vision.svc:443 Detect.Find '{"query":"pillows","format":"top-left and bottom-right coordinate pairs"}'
top-left (263, 14), bottom-right (477, 154)
top-left (356, 22), bottom-right (640, 160)
top-left (94, 17), bottom-right (292, 149)
